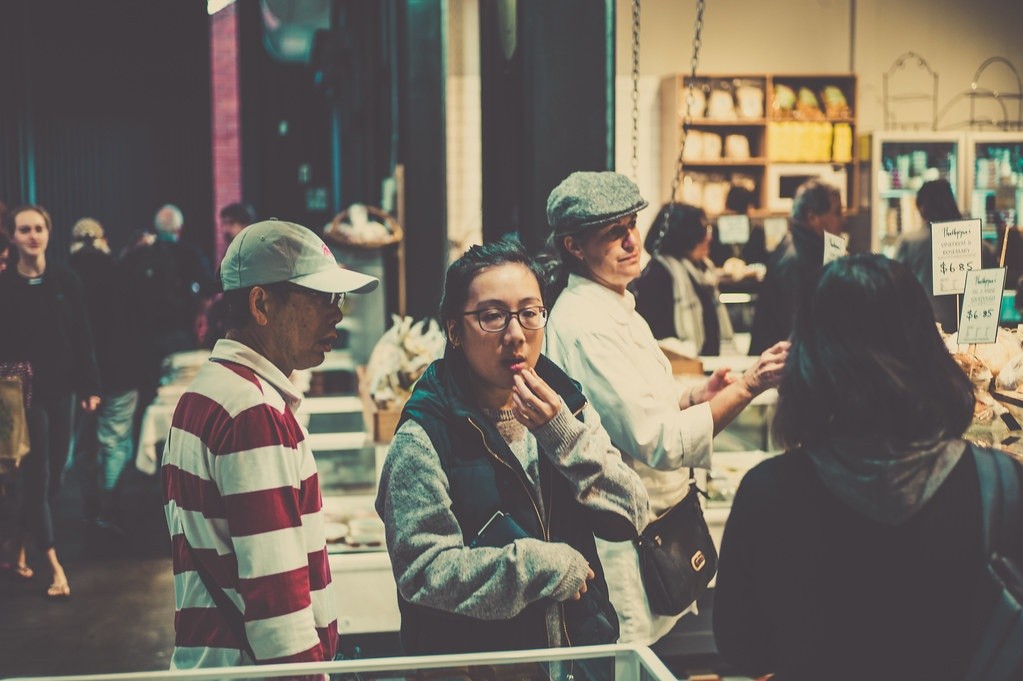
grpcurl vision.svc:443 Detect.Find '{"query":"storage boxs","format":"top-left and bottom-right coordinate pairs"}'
top-left (356, 364), bottom-right (413, 442)
top-left (768, 164), bottom-right (848, 213)
top-left (660, 345), bottom-right (705, 378)
top-left (766, 120), bottom-right (834, 163)
top-left (832, 122), bottom-right (854, 163)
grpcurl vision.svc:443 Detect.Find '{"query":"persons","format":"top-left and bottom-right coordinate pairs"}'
top-left (712, 252), bottom-right (1023, 681)
top-left (373, 239), bottom-right (652, 681)
top-left (540, 172), bottom-right (793, 647)
top-left (746, 180), bottom-right (849, 357)
top-left (160, 221), bottom-right (379, 681)
top-left (631, 202), bottom-right (732, 378)
top-left (0, 201), bottom-right (256, 597)
top-left (894, 180), bottom-right (998, 335)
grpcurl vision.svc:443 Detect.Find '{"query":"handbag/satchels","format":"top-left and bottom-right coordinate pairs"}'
top-left (465, 509), bottom-right (620, 681)
top-left (632, 485), bottom-right (721, 617)
top-left (0, 375), bottom-right (32, 465)
top-left (972, 439), bottom-right (1023, 681)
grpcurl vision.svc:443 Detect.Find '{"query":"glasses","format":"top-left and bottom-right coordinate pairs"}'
top-left (279, 289), bottom-right (347, 314)
top-left (462, 307), bottom-right (549, 332)
top-left (826, 207), bottom-right (842, 218)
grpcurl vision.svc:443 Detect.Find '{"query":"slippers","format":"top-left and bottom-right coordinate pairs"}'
top-left (12, 565), bottom-right (33, 578)
top-left (47, 582), bottom-right (69, 600)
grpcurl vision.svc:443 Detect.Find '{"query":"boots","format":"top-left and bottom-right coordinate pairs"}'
top-left (96, 489), bottom-right (132, 538)
top-left (80, 492), bottom-right (100, 524)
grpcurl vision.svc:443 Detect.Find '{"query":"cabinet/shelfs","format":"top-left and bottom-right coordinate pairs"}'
top-left (657, 71), bottom-right (861, 245)
top-left (872, 131), bottom-right (968, 257)
top-left (965, 132), bottom-right (1023, 232)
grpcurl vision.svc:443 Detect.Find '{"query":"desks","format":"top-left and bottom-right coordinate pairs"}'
top-left (696, 355), bottom-right (779, 452)
top-left (328, 551), bottom-right (402, 636)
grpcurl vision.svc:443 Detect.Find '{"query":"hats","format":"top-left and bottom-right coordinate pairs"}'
top-left (220, 216), bottom-right (380, 295)
top-left (71, 218), bottom-right (103, 240)
top-left (546, 171), bottom-right (648, 235)
